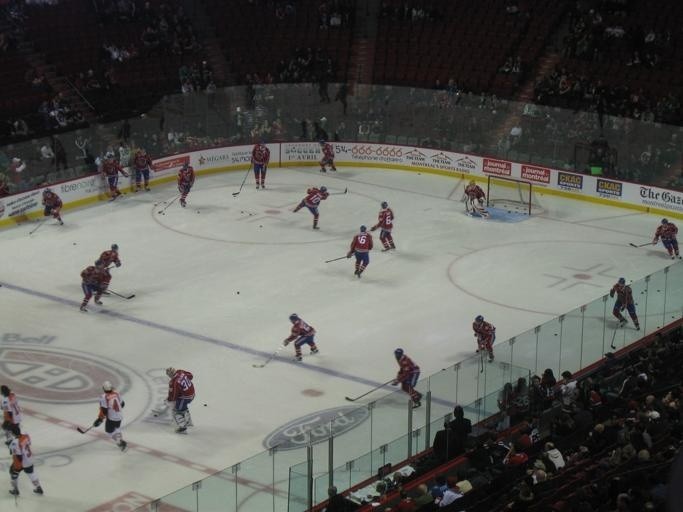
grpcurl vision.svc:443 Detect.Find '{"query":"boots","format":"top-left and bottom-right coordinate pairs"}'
top-left (354, 268), bottom-right (359, 275)
top-left (294, 354), bottom-right (302, 363)
top-left (186, 419), bottom-right (196, 428)
top-left (94, 298), bottom-right (103, 306)
top-left (389, 244), bottom-right (397, 253)
top-left (80, 305), bottom-right (88, 313)
top-left (380, 246), bottom-right (389, 255)
top-left (676, 252), bottom-right (682, 260)
top-left (488, 356), bottom-right (494, 363)
top-left (33, 487), bottom-right (44, 495)
top-left (180, 199), bottom-right (187, 208)
top-left (412, 392), bottom-right (422, 410)
top-left (174, 424), bottom-right (188, 435)
top-left (330, 166), bottom-right (336, 172)
top-left (357, 270), bottom-right (364, 280)
top-left (475, 348), bottom-right (481, 353)
top-left (57, 218), bottom-right (64, 227)
top-left (670, 252), bottom-right (675, 260)
top-left (9, 487), bottom-right (20, 497)
top-left (255, 183), bottom-right (260, 193)
top-left (117, 192), bottom-right (125, 201)
top-left (319, 168), bottom-right (327, 174)
top-left (107, 194), bottom-right (117, 204)
top-left (144, 184), bottom-right (150, 193)
top-left (620, 317), bottom-right (628, 328)
top-left (309, 348), bottom-right (319, 355)
top-left (292, 208), bottom-right (299, 214)
top-left (632, 320), bottom-right (642, 331)
top-left (118, 440), bottom-right (128, 454)
top-left (134, 187), bottom-right (141, 193)
top-left (261, 182), bottom-right (265, 190)
top-left (313, 220), bottom-right (320, 230)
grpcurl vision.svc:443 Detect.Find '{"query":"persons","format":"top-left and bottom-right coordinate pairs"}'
top-left (175, 58), bottom-right (217, 99)
top-left (610, 277), bottom-right (639, 331)
top-left (105, 144), bottom-right (115, 157)
top-left (1, 0), bottom-right (62, 16)
top-left (392, 349), bottom-right (423, 403)
top-left (379, 0), bottom-right (441, 24)
top-left (327, 392), bottom-right (683, 512)
top-left (293, 185), bottom-right (330, 230)
top-left (252, 137), bottom-right (270, 190)
top-left (134, 146), bottom-right (154, 192)
top-left (96, 151), bottom-right (108, 173)
top-left (666, 169), bottom-right (683, 192)
top-left (166, 367), bottom-right (195, 433)
top-left (1, 16), bottom-right (99, 190)
top-left (354, 78), bottom-right (496, 152)
top-left (103, 154), bottom-right (129, 202)
top-left (652, 217), bottom-right (682, 259)
top-left (318, 0), bottom-right (355, 32)
top-left (103, 41), bottom-right (138, 62)
top-left (283, 313), bottom-right (319, 361)
top-left (497, 325), bottom-right (683, 404)
top-left (177, 161), bottom-right (195, 208)
top-left (496, 0), bottom-right (682, 183)
top-left (97, 244), bottom-right (121, 295)
top-left (99, 65), bottom-right (123, 94)
top-left (319, 140), bottom-right (337, 173)
top-left (234, 45), bottom-right (341, 142)
top-left (42, 188), bottom-right (63, 226)
top-left (347, 226), bottom-right (373, 278)
top-left (0, 385), bottom-right (22, 445)
top-left (464, 181), bottom-right (491, 218)
top-left (272, 0), bottom-right (294, 19)
top-left (100, 0), bottom-right (198, 57)
top-left (370, 201), bottom-right (395, 251)
top-left (144, 124), bottom-right (225, 151)
top-left (8, 426), bottom-right (43, 496)
top-left (80, 259), bottom-right (106, 312)
top-left (93, 380), bottom-right (129, 453)
top-left (472, 314), bottom-right (495, 363)
top-left (118, 141), bottom-right (131, 168)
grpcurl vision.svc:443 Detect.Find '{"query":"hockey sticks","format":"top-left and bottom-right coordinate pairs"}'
top-left (480, 348), bottom-right (483, 373)
top-left (252, 344), bottom-right (285, 368)
top-left (77, 427), bottom-right (94, 434)
top-left (610, 321), bottom-right (619, 349)
top-left (325, 255), bottom-right (352, 263)
top-left (29, 214), bottom-right (51, 234)
top-left (345, 378), bottom-right (398, 402)
top-left (232, 161), bottom-right (252, 195)
top-left (328, 188), bottom-right (348, 195)
top-left (158, 194), bottom-right (180, 214)
top-left (628, 241), bottom-right (655, 248)
top-left (106, 288), bottom-right (135, 299)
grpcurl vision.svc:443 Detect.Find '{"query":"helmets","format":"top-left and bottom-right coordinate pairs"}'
top-left (475, 314), bottom-right (484, 325)
top-left (358, 225), bottom-right (366, 232)
top-left (0, 385), bottom-right (10, 395)
top-left (44, 188), bottom-right (51, 195)
top-left (661, 218), bottom-right (668, 224)
top-left (164, 366), bottom-right (176, 379)
top-left (394, 348), bottom-right (403, 359)
top-left (319, 186), bottom-right (327, 192)
top-left (7, 425), bottom-right (20, 436)
top-left (468, 179), bottom-right (476, 188)
top-left (380, 200), bottom-right (388, 211)
top-left (182, 163), bottom-right (188, 169)
top-left (111, 244), bottom-right (118, 252)
top-left (95, 259), bottom-right (105, 269)
top-left (107, 154), bottom-right (114, 161)
top-left (288, 313), bottom-right (299, 323)
top-left (102, 381), bottom-right (114, 394)
top-left (619, 278), bottom-right (625, 286)
top-left (318, 140), bottom-right (325, 145)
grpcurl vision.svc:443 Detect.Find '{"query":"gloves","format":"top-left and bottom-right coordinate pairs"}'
top-left (344, 251), bottom-right (354, 259)
top-left (620, 305), bottom-right (625, 311)
top-left (389, 379), bottom-right (400, 386)
top-left (369, 226), bottom-right (377, 232)
top-left (93, 419), bottom-right (102, 427)
top-left (652, 238), bottom-right (659, 245)
top-left (281, 339), bottom-right (289, 347)
top-left (610, 292), bottom-right (613, 298)
top-left (122, 171), bottom-right (129, 177)
top-left (114, 262), bottom-right (122, 268)
